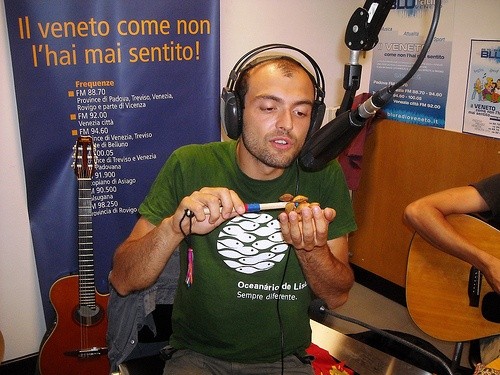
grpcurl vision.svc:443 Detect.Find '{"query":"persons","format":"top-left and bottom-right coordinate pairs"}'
top-left (108, 43), bottom-right (358, 375)
top-left (402, 174), bottom-right (500, 375)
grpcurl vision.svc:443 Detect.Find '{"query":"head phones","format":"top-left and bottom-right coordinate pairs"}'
top-left (221, 43), bottom-right (326, 140)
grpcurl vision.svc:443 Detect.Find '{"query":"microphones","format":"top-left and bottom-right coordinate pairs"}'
top-left (309, 299), bottom-right (454, 375)
top-left (299, 85), bottom-right (395, 172)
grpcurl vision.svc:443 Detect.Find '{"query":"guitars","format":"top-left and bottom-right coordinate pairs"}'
top-left (39, 135), bottom-right (111, 375)
top-left (406, 214), bottom-right (500, 343)
